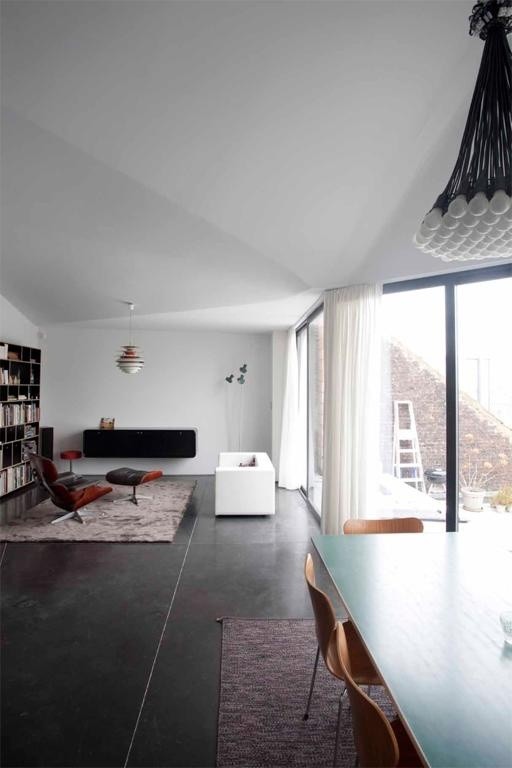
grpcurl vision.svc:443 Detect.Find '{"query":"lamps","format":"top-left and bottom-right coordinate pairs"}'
top-left (116, 305), bottom-right (144, 375)
top-left (225, 363), bottom-right (252, 452)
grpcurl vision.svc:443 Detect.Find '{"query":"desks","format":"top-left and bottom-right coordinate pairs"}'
top-left (309, 532), bottom-right (512, 767)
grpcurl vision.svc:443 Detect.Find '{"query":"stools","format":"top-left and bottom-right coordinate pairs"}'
top-left (60, 450), bottom-right (82, 474)
top-left (106, 466), bottom-right (162, 505)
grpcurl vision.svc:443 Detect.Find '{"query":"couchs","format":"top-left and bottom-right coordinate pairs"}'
top-left (215, 451), bottom-right (276, 519)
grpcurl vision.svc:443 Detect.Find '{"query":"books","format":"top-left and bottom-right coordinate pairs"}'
top-left (0, 343), bottom-right (40, 496)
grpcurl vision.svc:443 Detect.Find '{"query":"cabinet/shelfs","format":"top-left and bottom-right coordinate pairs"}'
top-left (83, 428), bottom-right (197, 458)
top-left (40, 427), bottom-right (56, 462)
top-left (0, 342), bottom-right (43, 507)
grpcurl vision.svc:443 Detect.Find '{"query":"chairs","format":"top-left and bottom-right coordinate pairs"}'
top-left (334, 619), bottom-right (435, 768)
top-left (301, 553), bottom-right (400, 719)
top-left (343, 518), bottom-right (425, 534)
top-left (29, 452), bottom-right (112, 523)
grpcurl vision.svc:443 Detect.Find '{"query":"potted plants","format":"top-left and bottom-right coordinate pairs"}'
top-left (457, 436), bottom-right (502, 512)
top-left (491, 486), bottom-right (512, 513)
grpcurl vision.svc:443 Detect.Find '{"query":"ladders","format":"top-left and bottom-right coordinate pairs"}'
top-left (393, 400), bottom-right (427, 495)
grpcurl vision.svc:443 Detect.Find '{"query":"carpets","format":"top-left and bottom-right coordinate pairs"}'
top-left (213, 618), bottom-right (401, 766)
top-left (0, 478), bottom-right (197, 542)
